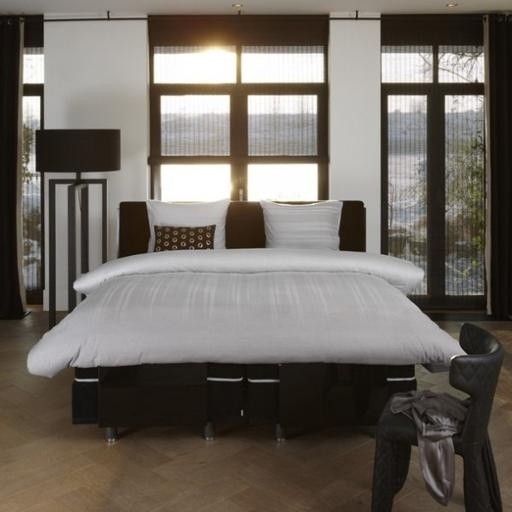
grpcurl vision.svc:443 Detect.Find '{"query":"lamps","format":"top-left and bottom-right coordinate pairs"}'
top-left (35, 129), bottom-right (121, 331)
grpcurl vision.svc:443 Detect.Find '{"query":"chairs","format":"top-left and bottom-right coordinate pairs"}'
top-left (371, 323), bottom-right (511, 510)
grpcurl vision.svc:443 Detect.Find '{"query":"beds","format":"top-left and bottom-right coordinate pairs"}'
top-left (71, 199), bottom-right (420, 444)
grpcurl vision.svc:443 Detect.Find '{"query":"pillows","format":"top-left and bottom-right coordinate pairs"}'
top-left (141, 201), bottom-right (347, 267)
